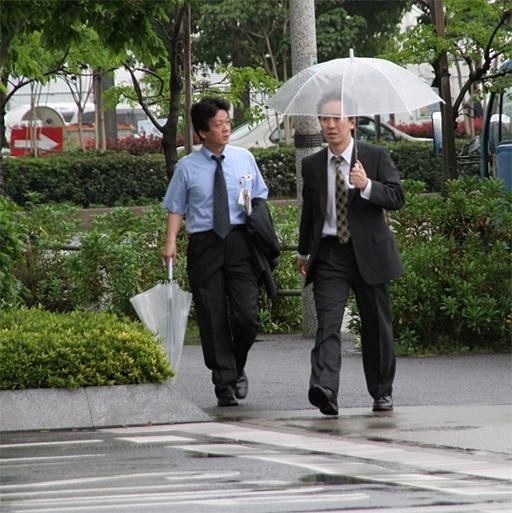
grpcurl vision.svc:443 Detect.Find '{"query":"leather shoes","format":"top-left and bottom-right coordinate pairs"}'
top-left (372, 394), bottom-right (394, 411)
top-left (231, 370), bottom-right (248, 399)
top-left (217, 395), bottom-right (238, 407)
top-left (307, 383), bottom-right (338, 415)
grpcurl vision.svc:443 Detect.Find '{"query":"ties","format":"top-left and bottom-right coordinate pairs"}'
top-left (210, 154), bottom-right (233, 240)
top-left (331, 154), bottom-right (352, 244)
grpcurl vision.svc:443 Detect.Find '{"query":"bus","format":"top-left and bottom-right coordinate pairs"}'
top-left (66, 122), bottom-right (142, 150)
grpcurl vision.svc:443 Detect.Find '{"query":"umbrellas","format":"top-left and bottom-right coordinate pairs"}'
top-left (268, 49), bottom-right (440, 189)
top-left (129, 252), bottom-right (191, 388)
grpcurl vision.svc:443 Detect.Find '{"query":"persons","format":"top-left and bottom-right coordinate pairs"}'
top-left (293, 91), bottom-right (408, 416)
top-left (161, 97), bottom-right (280, 408)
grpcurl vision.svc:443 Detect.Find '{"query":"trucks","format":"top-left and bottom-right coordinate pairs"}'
top-left (432, 57), bottom-right (512, 194)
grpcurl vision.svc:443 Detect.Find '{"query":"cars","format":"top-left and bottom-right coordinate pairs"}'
top-left (176, 114), bottom-right (433, 152)
